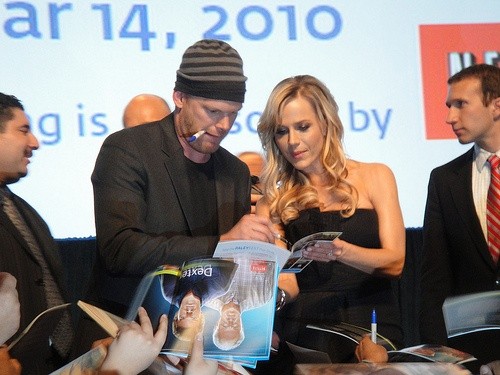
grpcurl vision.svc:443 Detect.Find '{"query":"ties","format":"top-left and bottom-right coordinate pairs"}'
top-left (487, 155), bottom-right (500, 262)
top-left (0, 187), bottom-right (75, 359)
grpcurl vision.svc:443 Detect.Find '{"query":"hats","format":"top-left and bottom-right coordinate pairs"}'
top-left (174, 39), bottom-right (247, 104)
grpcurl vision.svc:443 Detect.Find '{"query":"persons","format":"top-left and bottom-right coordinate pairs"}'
top-left (417, 63), bottom-right (500, 375)
top-left (354, 334), bottom-right (388, 364)
top-left (0, 93), bottom-right (219, 375)
top-left (91, 40), bottom-right (279, 375)
top-left (173, 256), bottom-right (275, 351)
top-left (256, 74), bottom-right (405, 375)
top-left (237, 152), bottom-right (265, 214)
top-left (414, 342), bottom-right (468, 359)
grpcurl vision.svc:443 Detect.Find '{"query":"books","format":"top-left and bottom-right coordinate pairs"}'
top-left (304, 319), bottom-right (477, 366)
top-left (78, 259), bottom-right (280, 369)
top-left (443, 290), bottom-right (500, 338)
top-left (214, 232), bottom-right (342, 273)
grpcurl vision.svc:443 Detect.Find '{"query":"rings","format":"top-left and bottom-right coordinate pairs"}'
top-left (328, 250), bottom-right (333, 256)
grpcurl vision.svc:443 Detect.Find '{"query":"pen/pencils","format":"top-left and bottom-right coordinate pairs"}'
top-left (372, 309), bottom-right (377, 344)
top-left (189, 130), bottom-right (206, 143)
top-left (270, 232), bottom-right (291, 244)
